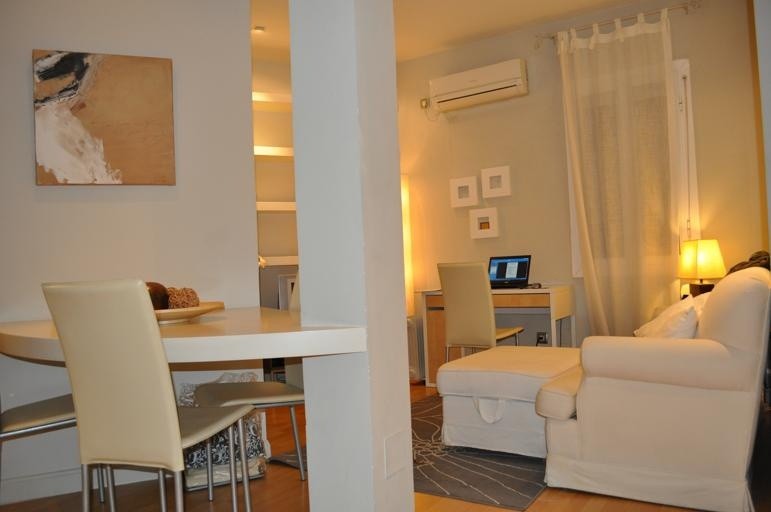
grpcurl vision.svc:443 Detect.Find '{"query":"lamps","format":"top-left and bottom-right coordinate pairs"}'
top-left (674, 240), bottom-right (727, 297)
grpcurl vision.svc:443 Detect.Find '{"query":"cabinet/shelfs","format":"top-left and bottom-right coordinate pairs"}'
top-left (254, 93), bottom-right (300, 381)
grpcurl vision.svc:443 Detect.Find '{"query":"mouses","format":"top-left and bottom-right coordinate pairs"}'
top-left (532, 283), bottom-right (542, 288)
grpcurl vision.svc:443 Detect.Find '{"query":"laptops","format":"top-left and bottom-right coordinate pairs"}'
top-left (488, 255), bottom-right (532, 289)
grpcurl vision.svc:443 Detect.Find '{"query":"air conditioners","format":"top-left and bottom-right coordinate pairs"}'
top-left (430, 57), bottom-right (528, 113)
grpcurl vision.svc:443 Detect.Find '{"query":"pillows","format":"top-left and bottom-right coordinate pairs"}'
top-left (634, 293), bottom-right (700, 339)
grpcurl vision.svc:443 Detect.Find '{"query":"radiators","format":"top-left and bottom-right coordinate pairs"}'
top-left (407, 314), bottom-right (425, 384)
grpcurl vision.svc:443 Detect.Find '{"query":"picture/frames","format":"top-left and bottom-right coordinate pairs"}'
top-left (480, 165), bottom-right (510, 197)
top-left (277, 274), bottom-right (298, 311)
top-left (468, 207), bottom-right (501, 239)
top-left (449, 177), bottom-right (478, 209)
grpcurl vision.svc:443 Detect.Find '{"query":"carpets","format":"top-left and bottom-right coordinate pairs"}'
top-left (268, 393), bottom-right (549, 512)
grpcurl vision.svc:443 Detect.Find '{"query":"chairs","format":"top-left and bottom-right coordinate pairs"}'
top-left (43, 279), bottom-right (253, 512)
top-left (437, 262), bottom-right (525, 364)
top-left (1, 393), bottom-right (116, 512)
top-left (534, 266), bottom-right (771, 511)
top-left (192, 263), bottom-right (306, 512)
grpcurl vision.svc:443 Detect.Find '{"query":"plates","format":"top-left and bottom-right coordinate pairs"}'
top-left (154, 301), bottom-right (226, 326)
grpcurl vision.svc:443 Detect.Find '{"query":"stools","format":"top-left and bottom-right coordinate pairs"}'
top-left (436, 346), bottom-right (582, 458)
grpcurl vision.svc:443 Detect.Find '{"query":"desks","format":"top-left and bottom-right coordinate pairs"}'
top-left (423, 283), bottom-right (575, 390)
top-left (0, 307), bottom-right (366, 511)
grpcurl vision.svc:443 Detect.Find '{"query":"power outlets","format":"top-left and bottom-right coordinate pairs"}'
top-left (537, 332), bottom-right (548, 344)
top-left (420, 97), bottom-right (428, 109)
